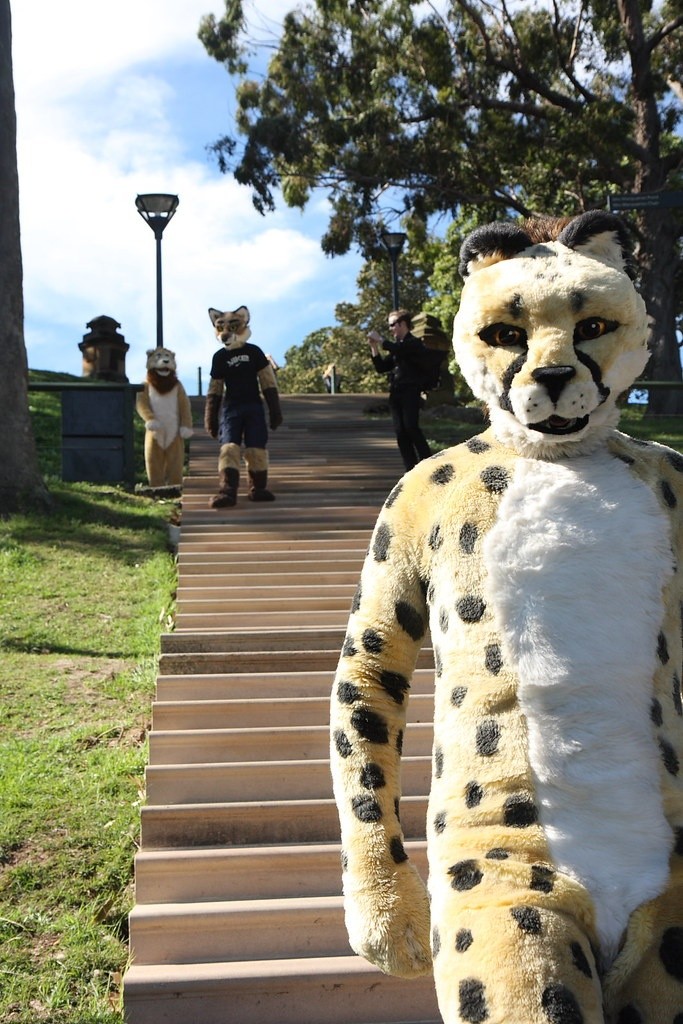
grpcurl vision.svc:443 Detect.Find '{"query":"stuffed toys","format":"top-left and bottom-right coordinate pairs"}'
top-left (205, 306), bottom-right (283, 509)
top-left (329, 209), bottom-right (682, 1023)
top-left (135, 347), bottom-right (193, 488)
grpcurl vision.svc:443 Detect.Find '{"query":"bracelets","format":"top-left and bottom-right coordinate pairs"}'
top-left (379, 337), bottom-right (382, 341)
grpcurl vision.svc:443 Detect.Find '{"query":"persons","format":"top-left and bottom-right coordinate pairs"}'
top-left (265, 354), bottom-right (279, 377)
top-left (367, 309), bottom-right (431, 469)
top-left (324, 363), bottom-right (341, 394)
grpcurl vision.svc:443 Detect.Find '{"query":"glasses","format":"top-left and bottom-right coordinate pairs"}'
top-left (389, 321), bottom-right (398, 328)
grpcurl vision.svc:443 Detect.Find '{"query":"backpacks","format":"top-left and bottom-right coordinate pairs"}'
top-left (404, 337), bottom-right (450, 390)
